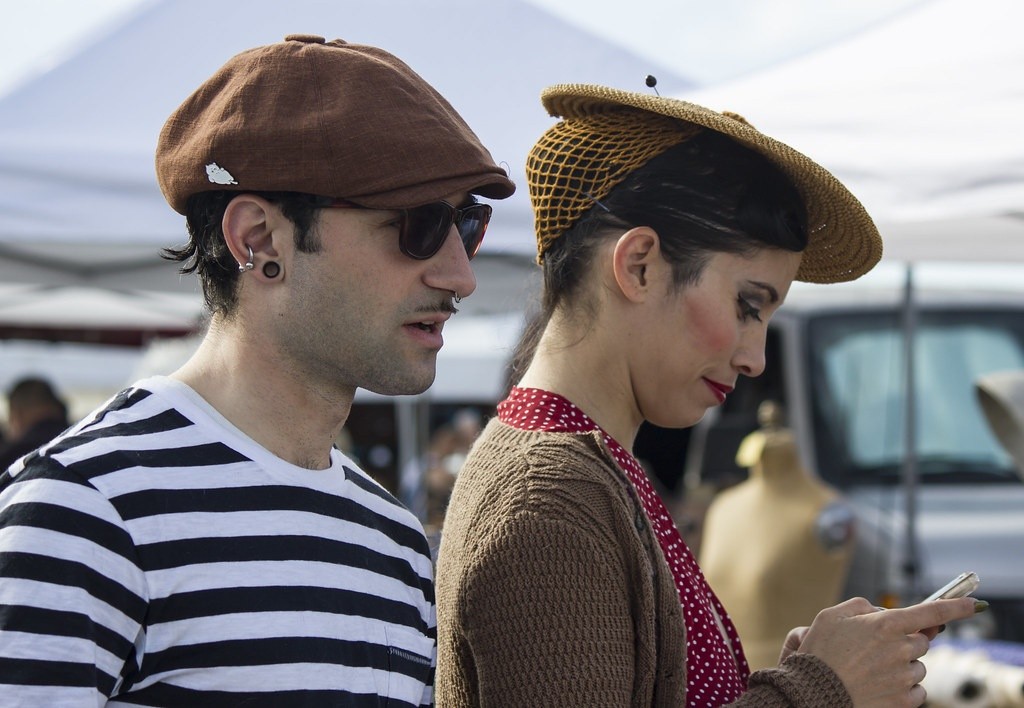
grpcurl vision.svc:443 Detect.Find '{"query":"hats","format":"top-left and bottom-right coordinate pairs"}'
top-left (155, 34), bottom-right (516, 210)
top-left (526, 83), bottom-right (883, 281)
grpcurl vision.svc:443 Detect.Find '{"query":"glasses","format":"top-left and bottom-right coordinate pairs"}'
top-left (312, 199), bottom-right (493, 262)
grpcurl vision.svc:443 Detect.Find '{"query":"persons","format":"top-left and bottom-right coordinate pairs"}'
top-left (0, 379), bottom-right (77, 472)
top-left (0, 33), bottom-right (514, 708)
top-left (434, 83), bottom-right (976, 708)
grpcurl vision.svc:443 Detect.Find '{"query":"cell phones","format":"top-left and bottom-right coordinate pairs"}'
top-left (922, 571), bottom-right (980, 603)
